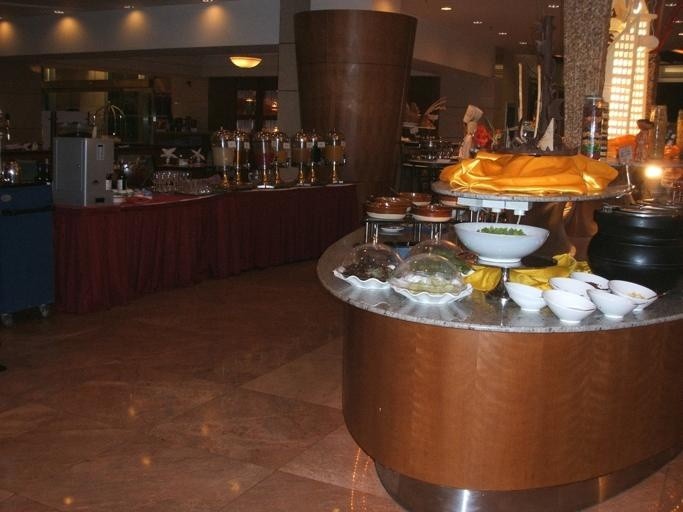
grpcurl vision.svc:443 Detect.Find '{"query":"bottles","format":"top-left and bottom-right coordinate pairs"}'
top-left (581, 103), bottom-right (683, 164)
top-left (0, 113), bottom-right (51, 186)
top-left (153, 115), bottom-right (210, 198)
top-left (116, 162), bottom-right (127, 190)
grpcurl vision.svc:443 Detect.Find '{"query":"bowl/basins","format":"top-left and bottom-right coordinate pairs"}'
top-left (607, 278), bottom-right (658, 313)
top-left (543, 290), bottom-right (595, 321)
top-left (502, 280), bottom-right (547, 311)
top-left (586, 290), bottom-right (638, 320)
top-left (569, 270), bottom-right (609, 291)
top-left (548, 277), bottom-right (596, 303)
top-left (452, 220), bottom-right (551, 267)
top-left (363, 190), bottom-right (464, 223)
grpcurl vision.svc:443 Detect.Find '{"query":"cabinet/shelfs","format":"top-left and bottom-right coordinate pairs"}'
top-left (106, 87), bottom-right (173, 146)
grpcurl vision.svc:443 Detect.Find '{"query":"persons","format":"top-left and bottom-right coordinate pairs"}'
top-left (462, 118), bottom-right (493, 152)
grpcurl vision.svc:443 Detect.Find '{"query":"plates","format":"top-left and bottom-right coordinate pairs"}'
top-left (332, 259), bottom-right (476, 305)
top-left (379, 226), bottom-right (405, 233)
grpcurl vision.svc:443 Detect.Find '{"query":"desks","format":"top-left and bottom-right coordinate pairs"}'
top-left (49, 181), bottom-right (361, 315)
top-left (402, 157), bottom-right (460, 192)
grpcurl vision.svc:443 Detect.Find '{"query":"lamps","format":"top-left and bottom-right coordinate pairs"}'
top-left (229, 55), bottom-right (264, 70)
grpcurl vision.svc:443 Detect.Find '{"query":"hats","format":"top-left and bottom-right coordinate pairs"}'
top-left (463, 104), bottom-right (483, 124)
